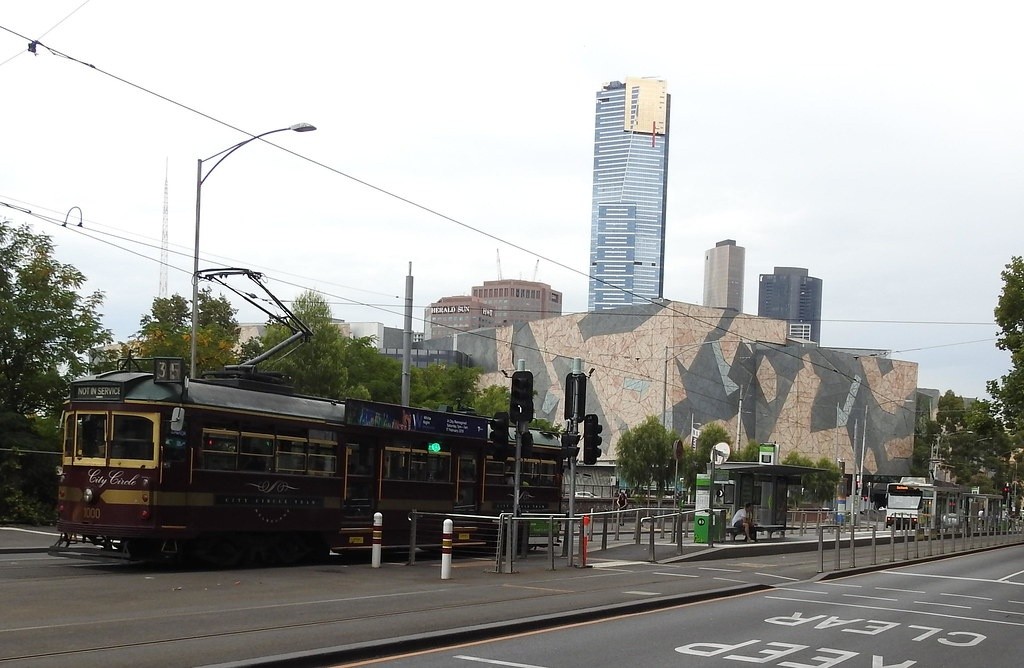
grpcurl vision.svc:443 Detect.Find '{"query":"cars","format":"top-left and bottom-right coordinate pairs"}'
top-left (575, 491), bottom-right (600, 499)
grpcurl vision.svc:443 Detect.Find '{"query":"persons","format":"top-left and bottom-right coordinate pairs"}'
top-left (732, 502), bottom-right (755, 542)
top-left (978, 508), bottom-right (985, 527)
top-left (494, 464), bottom-right (511, 484)
top-left (617, 492), bottom-right (628, 526)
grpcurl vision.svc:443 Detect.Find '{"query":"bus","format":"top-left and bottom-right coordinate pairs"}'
top-left (47, 265), bottom-right (564, 574)
top-left (885, 476), bottom-right (963, 530)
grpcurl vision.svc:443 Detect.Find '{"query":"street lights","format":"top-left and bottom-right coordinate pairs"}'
top-left (189, 123), bottom-right (317, 381)
top-left (858, 399), bottom-right (915, 532)
top-left (656, 340), bottom-right (757, 529)
top-left (928, 431), bottom-right (973, 476)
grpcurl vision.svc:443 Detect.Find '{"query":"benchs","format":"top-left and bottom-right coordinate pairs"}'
top-left (725, 524), bottom-right (785, 541)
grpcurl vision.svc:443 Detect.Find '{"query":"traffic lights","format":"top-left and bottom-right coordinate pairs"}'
top-left (561, 432), bottom-right (581, 460)
top-left (584, 414), bottom-right (602, 465)
top-left (1003, 487), bottom-right (1008, 493)
top-left (486, 412), bottom-right (509, 461)
top-left (511, 371), bottom-right (533, 425)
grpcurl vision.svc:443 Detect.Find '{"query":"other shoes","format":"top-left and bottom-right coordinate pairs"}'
top-left (747, 539), bottom-right (755, 543)
